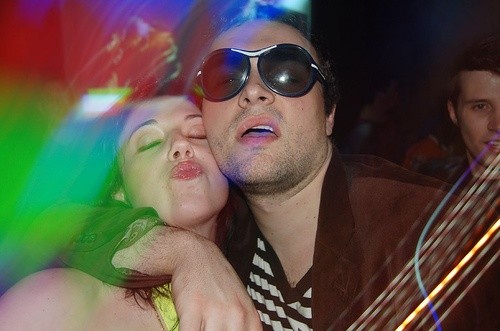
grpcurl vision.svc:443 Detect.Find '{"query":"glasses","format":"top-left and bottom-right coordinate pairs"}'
top-left (194, 43), bottom-right (327, 101)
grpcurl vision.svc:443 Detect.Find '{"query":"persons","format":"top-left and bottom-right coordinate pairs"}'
top-left (25, 4), bottom-right (500, 331)
top-left (420, 44), bottom-right (500, 212)
top-left (351, 72), bottom-right (405, 166)
top-left (1, 76), bottom-right (249, 331)
top-left (404, 100), bottom-right (462, 183)
